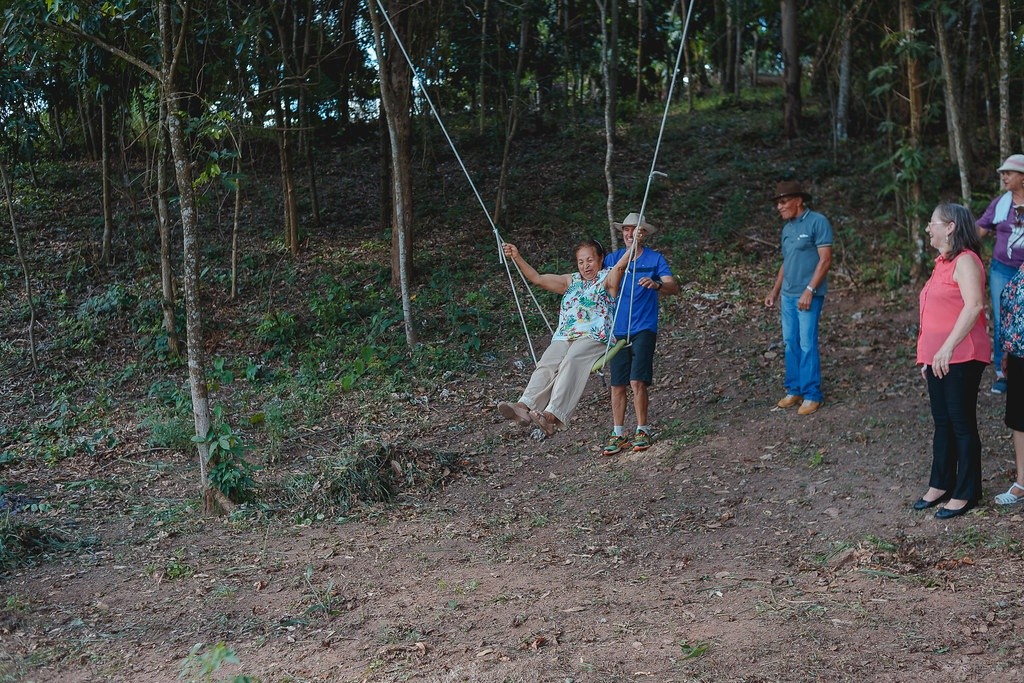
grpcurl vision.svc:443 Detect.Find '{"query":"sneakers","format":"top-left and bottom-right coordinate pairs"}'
top-left (798, 397), bottom-right (825, 415)
top-left (602, 433), bottom-right (630, 457)
top-left (777, 392), bottom-right (801, 409)
top-left (633, 428), bottom-right (653, 451)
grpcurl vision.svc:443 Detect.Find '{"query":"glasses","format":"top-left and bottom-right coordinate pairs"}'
top-left (769, 198), bottom-right (798, 205)
top-left (926, 221), bottom-right (949, 230)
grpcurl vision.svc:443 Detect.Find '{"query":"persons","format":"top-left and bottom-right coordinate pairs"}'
top-left (497, 227), bottom-right (646, 439)
top-left (994, 264), bottom-right (1024, 504)
top-left (976, 154), bottom-right (1024, 394)
top-left (765, 181), bottom-right (834, 416)
top-left (912, 203), bottom-right (992, 520)
top-left (603, 212), bottom-right (676, 456)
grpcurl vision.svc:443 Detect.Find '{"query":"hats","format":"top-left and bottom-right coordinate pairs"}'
top-left (997, 153), bottom-right (1024, 173)
top-left (772, 181), bottom-right (812, 202)
top-left (612, 211), bottom-right (658, 236)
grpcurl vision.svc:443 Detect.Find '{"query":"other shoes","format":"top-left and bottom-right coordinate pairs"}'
top-left (934, 498), bottom-right (979, 520)
top-left (498, 401), bottom-right (532, 427)
top-left (913, 488), bottom-right (953, 510)
top-left (528, 409), bottom-right (558, 437)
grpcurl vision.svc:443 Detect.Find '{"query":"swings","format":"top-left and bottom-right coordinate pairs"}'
top-left (373, 0), bottom-right (699, 376)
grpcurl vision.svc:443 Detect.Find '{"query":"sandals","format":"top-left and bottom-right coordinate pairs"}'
top-left (994, 481), bottom-right (1024, 506)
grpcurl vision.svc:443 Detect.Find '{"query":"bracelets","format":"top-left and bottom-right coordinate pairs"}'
top-left (655, 281), bottom-right (663, 291)
top-left (807, 285), bottom-right (816, 294)
